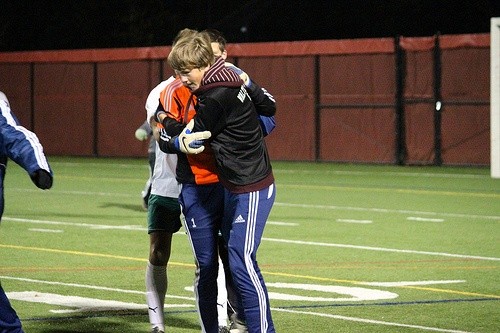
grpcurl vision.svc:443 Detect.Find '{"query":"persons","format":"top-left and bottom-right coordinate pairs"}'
top-left (0, 90), bottom-right (52, 333)
top-left (145, 31), bottom-right (228, 333)
top-left (135, 120), bottom-right (156, 210)
top-left (157, 29), bottom-right (276, 333)
top-left (153, 34), bottom-right (276, 333)
top-left (206, 32), bottom-right (243, 71)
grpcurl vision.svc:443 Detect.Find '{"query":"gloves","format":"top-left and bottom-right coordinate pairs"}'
top-left (170, 119), bottom-right (211, 155)
top-left (32, 170), bottom-right (51, 189)
top-left (224, 62), bottom-right (251, 88)
top-left (154, 98), bottom-right (166, 122)
